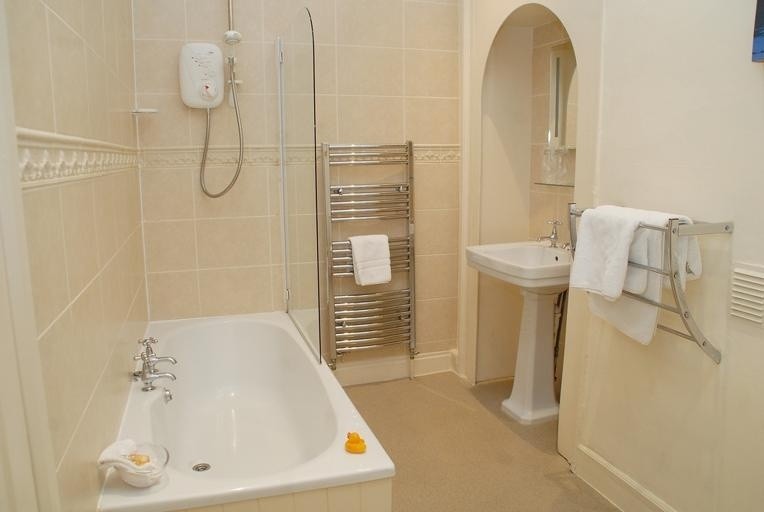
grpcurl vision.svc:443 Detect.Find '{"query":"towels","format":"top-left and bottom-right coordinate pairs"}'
top-left (346, 233), bottom-right (392, 287)
top-left (566, 204), bottom-right (703, 349)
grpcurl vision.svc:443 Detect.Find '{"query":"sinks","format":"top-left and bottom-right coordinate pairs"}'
top-left (466, 240), bottom-right (573, 280)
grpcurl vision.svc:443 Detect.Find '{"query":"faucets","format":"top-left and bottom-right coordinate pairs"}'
top-left (538, 218), bottom-right (563, 248)
top-left (133, 354), bottom-right (176, 392)
top-left (137, 338), bottom-right (177, 372)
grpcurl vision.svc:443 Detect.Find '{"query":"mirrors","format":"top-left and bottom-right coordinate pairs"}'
top-left (529, 20), bottom-right (578, 189)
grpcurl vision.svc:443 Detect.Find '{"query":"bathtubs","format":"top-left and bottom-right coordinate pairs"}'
top-left (94, 310), bottom-right (395, 511)
